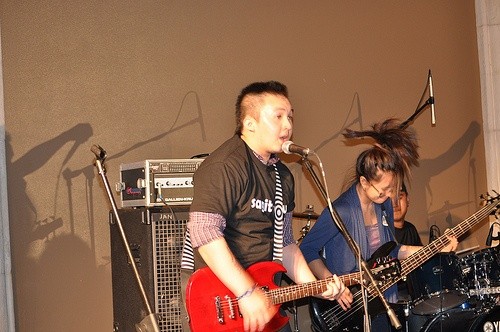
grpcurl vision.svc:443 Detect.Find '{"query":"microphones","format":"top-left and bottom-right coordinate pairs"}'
top-left (428, 76), bottom-right (435, 124)
top-left (485, 224), bottom-right (493, 246)
top-left (281, 141), bottom-right (310, 156)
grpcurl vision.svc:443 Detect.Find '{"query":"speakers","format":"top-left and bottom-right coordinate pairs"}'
top-left (111, 208), bottom-right (190, 332)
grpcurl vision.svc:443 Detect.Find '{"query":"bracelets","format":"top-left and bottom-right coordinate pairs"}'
top-left (237, 282), bottom-right (259, 301)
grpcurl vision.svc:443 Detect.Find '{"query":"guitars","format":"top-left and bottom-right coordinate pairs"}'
top-left (185, 255), bottom-right (404, 332)
top-left (308, 188), bottom-right (500, 332)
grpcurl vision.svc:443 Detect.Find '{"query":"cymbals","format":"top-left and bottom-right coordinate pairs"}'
top-left (291, 210), bottom-right (321, 220)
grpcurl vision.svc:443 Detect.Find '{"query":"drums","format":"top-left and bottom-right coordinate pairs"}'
top-left (420, 306), bottom-right (500, 332)
top-left (404, 248), bottom-right (470, 316)
top-left (460, 243), bottom-right (500, 297)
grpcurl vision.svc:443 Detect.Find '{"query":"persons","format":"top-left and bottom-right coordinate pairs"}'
top-left (393, 182), bottom-right (424, 246)
top-left (188, 81), bottom-right (345, 332)
top-left (298, 115), bottom-right (458, 332)
